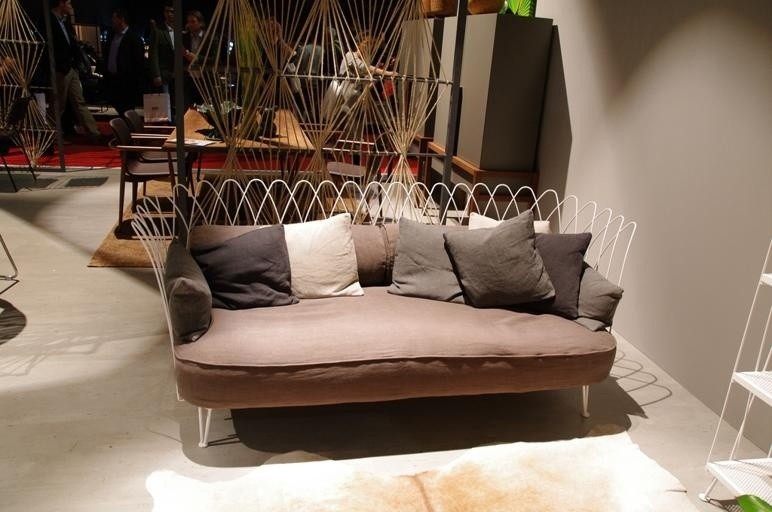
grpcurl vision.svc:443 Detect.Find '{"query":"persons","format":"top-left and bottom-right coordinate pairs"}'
top-left (38, 1), bottom-right (399, 146)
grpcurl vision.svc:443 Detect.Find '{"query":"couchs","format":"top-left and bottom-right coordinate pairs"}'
top-left (130, 178), bottom-right (636, 448)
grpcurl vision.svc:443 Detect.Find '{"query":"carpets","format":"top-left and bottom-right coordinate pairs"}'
top-left (88, 178), bottom-right (370, 268)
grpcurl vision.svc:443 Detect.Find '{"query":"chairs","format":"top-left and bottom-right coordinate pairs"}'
top-left (1, 98), bottom-right (36, 194)
top-left (108, 107), bottom-right (388, 232)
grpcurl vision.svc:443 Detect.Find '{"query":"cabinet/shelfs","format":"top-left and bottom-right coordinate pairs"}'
top-left (699, 236), bottom-right (772, 512)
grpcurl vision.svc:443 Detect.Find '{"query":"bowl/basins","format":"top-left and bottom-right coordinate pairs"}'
top-left (196, 100), bottom-right (246, 130)
